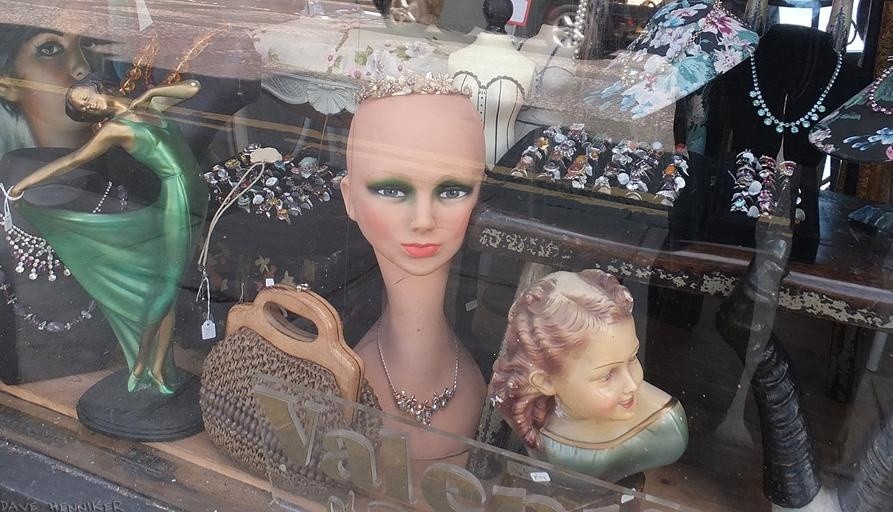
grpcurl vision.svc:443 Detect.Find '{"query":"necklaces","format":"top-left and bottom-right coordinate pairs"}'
top-left (0, 176), bottom-right (132, 340)
top-left (866, 51), bottom-right (891, 119)
top-left (614, 0), bottom-right (754, 100)
top-left (374, 314), bottom-right (462, 430)
top-left (113, 14), bottom-right (253, 104)
top-left (745, 39), bottom-right (844, 137)
top-left (572, 0), bottom-right (587, 63)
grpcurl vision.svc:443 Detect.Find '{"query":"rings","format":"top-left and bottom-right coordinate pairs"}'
top-left (200, 137), bottom-right (347, 227)
top-left (508, 120), bottom-right (693, 209)
top-left (727, 149), bottom-right (811, 226)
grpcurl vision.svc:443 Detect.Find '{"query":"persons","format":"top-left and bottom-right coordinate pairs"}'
top-left (6, 76), bottom-right (212, 399)
top-left (337, 68), bottom-right (489, 464)
top-left (1, 1), bottom-right (114, 153)
top-left (485, 264), bottom-right (694, 476)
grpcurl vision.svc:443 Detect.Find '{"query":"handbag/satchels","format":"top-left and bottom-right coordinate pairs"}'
top-left (198, 282), bottom-right (382, 510)
